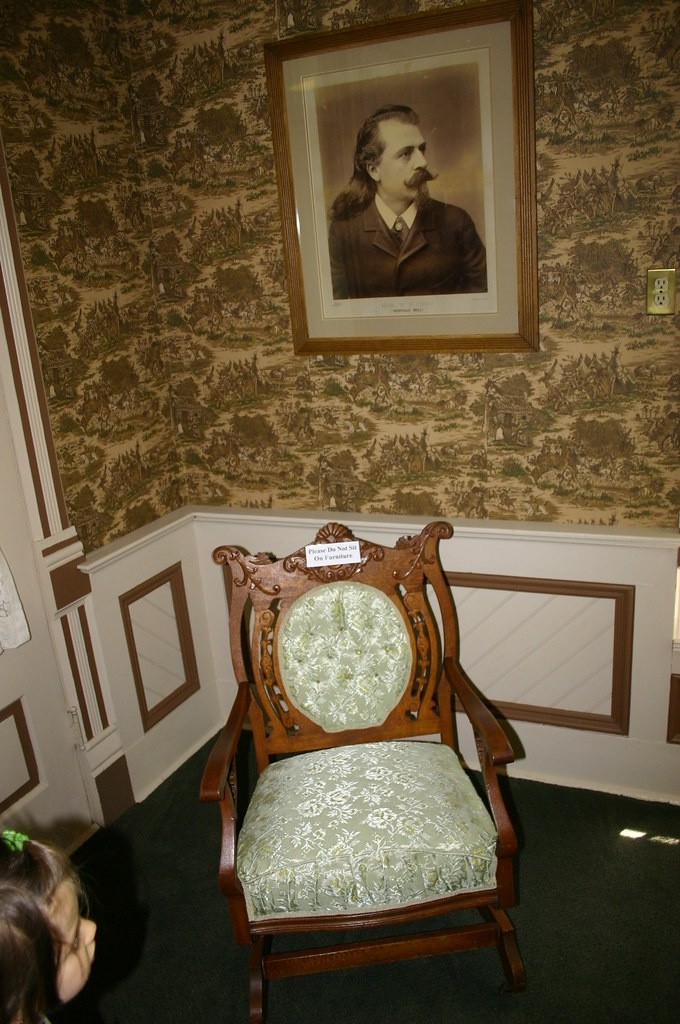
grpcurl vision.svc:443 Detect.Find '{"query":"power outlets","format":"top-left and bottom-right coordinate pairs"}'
top-left (646, 268), bottom-right (676, 315)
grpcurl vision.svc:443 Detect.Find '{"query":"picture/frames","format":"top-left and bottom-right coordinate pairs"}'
top-left (261, 0), bottom-right (534, 352)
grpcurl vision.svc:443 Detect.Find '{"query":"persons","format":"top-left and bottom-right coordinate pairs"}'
top-left (329, 104), bottom-right (488, 301)
top-left (1, 830), bottom-right (96, 1024)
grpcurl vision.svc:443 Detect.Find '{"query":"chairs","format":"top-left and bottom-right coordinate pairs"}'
top-left (199, 519), bottom-right (530, 1024)
